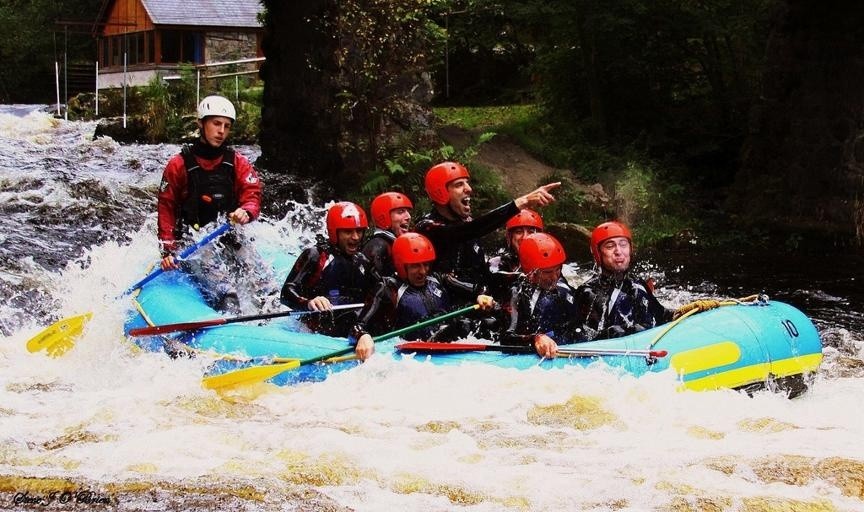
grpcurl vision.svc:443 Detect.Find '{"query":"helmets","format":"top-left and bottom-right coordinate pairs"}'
top-left (519, 232), bottom-right (566, 283)
top-left (197, 95), bottom-right (236, 124)
top-left (591, 220), bottom-right (633, 266)
top-left (392, 231), bottom-right (437, 280)
top-left (505, 208), bottom-right (544, 241)
top-left (326, 202), bottom-right (369, 245)
top-left (425, 161), bottom-right (471, 206)
top-left (370, 190), bottom-right (413, 230)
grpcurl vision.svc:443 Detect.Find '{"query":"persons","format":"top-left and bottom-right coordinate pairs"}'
top-left (500, 233), bottom-right (576, 360)
top-left (281, 201), bottom-right (368, 337)
top-left (359, 192), bottom-right (413, 277)
top-left (574, 222), bottom-right (673, 342)
top-left (411, 162), bottom-right (561, 287)
top-left (349, 233), bottom-right (496, 360)
top-left (483, 208), bottom-right (545, 305)
top-left (157, 95), bottom-right (281, 327)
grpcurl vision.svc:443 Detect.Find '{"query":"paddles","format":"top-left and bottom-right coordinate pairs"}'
top-left (202, 299), bottom-right (487, 397)
top-left (394, 341), bottom-right (668, 359)
top-left (26, 216), bottom-right (237, 361)
top-left (128, 301), bottom-right (365, 338)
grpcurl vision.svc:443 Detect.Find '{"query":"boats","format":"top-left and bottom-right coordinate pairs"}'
top-left (122, 248), bottom-right (823, 399)
